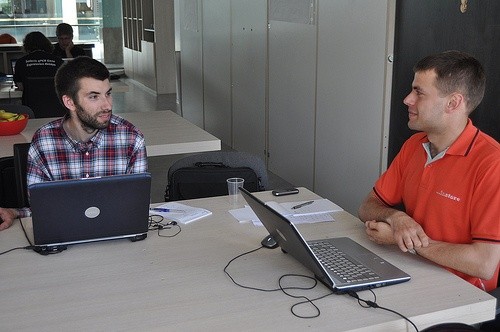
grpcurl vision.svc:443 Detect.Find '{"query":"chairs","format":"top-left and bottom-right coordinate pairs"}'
top-left (167, 151), bottom-right (272, 192)
top-left (0, 33), bottom-right (17, 44)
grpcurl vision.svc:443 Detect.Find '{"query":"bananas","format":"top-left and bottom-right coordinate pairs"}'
top-left (0, 109), bottom-right (20, 122)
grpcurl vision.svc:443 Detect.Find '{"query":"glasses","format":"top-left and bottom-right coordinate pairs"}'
top-left (58, 36), bottom-right (72, 42)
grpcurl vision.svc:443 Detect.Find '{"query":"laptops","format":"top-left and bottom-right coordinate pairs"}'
top-left (11, 60), bottom-right (17, 74)
top-left (238, 186), bottom-right (411, 293)
top-left (27, 173), bottom-right (153, 251)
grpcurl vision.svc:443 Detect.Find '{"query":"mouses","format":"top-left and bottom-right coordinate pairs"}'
top-left (261, 235), bottom-right (279, 249)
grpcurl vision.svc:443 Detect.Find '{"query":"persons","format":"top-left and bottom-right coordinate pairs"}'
top-left (359, 49), bottom-right (500, 294)
top-left (0, 56), bottom-right (148, 230)
top-left (13, 23), bottom-right (85, 118)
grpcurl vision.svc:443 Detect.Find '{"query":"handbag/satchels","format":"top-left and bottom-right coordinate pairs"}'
top-left (164, 162), bottom-right (265, 202)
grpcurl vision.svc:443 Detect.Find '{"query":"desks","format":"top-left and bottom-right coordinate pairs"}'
top-left (0, 186), bottom-right (497, 332)
top-left (0, 76), bottom-right (23, 98)
top-left (0, 40), bottom-right (95, 75)
top-left (0, 110), bottom-right (222, 158)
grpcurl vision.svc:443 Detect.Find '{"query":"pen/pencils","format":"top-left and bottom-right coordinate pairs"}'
top-left (293, 201), bottom-right (315, 209)
top-left (152, 208), bottom-right (184, 213)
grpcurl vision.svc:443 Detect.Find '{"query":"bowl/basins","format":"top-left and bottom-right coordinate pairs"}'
top-left (0, 113), bottom-right (29, 136)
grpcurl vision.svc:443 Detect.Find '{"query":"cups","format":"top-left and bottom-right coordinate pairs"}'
top-left (227, 177), bottom-right (244, 202)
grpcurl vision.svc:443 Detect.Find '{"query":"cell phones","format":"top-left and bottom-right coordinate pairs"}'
top-left (272, 189), bottom-right (299, 196)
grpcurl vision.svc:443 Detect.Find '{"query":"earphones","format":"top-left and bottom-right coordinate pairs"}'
top-left (157, 225), bottom-right (164, 230)
top-left (168, 221), bottom-right (177, 225)
